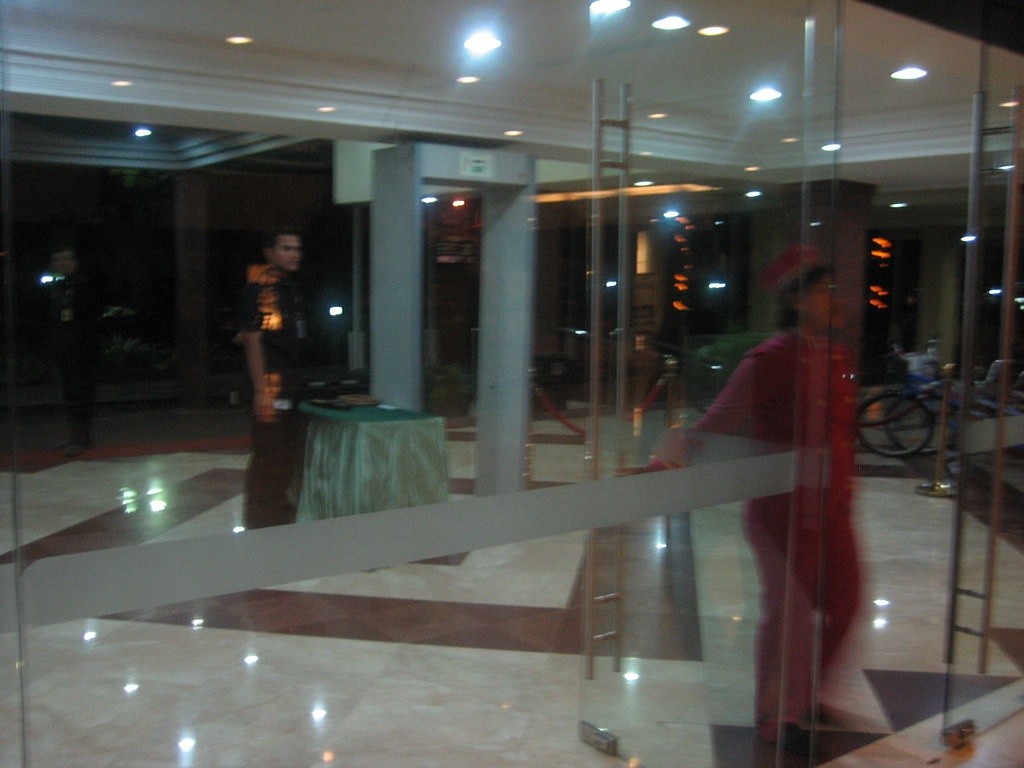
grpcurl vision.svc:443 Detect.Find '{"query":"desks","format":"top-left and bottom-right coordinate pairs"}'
top-left (293, 400), bottom-right (447, 518)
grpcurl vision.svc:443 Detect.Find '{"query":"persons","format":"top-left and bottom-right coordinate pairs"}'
top-left (236, 226), bottom-right (311, 529)
top-left (48, 245), bottom-right (101, 456)
top-left (652, 243), bottom-right (869, 757)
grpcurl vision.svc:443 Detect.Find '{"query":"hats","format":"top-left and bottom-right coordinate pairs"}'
top-left (755, 243), bottom-right (828, 295)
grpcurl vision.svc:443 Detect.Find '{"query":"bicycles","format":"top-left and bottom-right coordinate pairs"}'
top-left (853, 361), bottom-right (1002, 460)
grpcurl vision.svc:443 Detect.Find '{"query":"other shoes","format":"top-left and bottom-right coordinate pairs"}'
top-left (805, 704), bottom-right (840, 728)
top-left (782, 720), bottom-right (826, 760)
